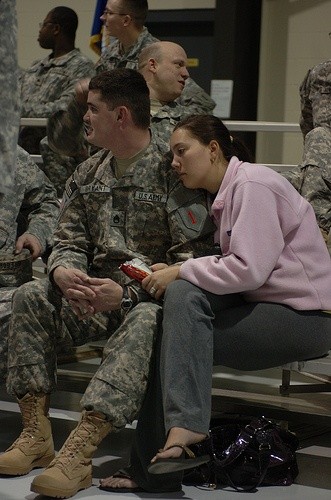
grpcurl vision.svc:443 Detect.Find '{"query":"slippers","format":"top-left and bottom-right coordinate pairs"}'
top-left (147, 443), bottom-right (211, 473)
top-left (98, 463), bottom-right (184, 493)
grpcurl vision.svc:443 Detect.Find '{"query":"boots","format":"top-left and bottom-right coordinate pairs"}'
top-left (30, 410), bottom-right (114, 499)
top-left (0, 391), bottom-right (55, 476)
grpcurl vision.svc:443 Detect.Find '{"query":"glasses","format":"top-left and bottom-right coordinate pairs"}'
top-left (38, 20), bottom-right (57, 28)
top-left (103, 9), bottom-right (128, 17)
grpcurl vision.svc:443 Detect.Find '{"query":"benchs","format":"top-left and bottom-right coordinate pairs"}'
top-left (48, 338), bottom-right (331, 416)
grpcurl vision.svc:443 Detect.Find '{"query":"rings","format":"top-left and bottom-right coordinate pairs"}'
top-left (150, 287), bottom-right (156, 294)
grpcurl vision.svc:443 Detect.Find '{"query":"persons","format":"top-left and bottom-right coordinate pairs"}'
top-left (0, 0), bottom-right (331, 382)
top-left (0, 68), bottom-right (216, 499)
top-left (99, 113), bottom-right (331, 491)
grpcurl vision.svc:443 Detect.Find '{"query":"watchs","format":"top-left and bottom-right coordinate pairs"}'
top-left (120, 286), bottom-right (133, 319)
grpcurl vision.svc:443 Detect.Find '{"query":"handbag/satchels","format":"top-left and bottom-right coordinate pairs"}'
top-left (197, 415), bottom-right (299, 493)
